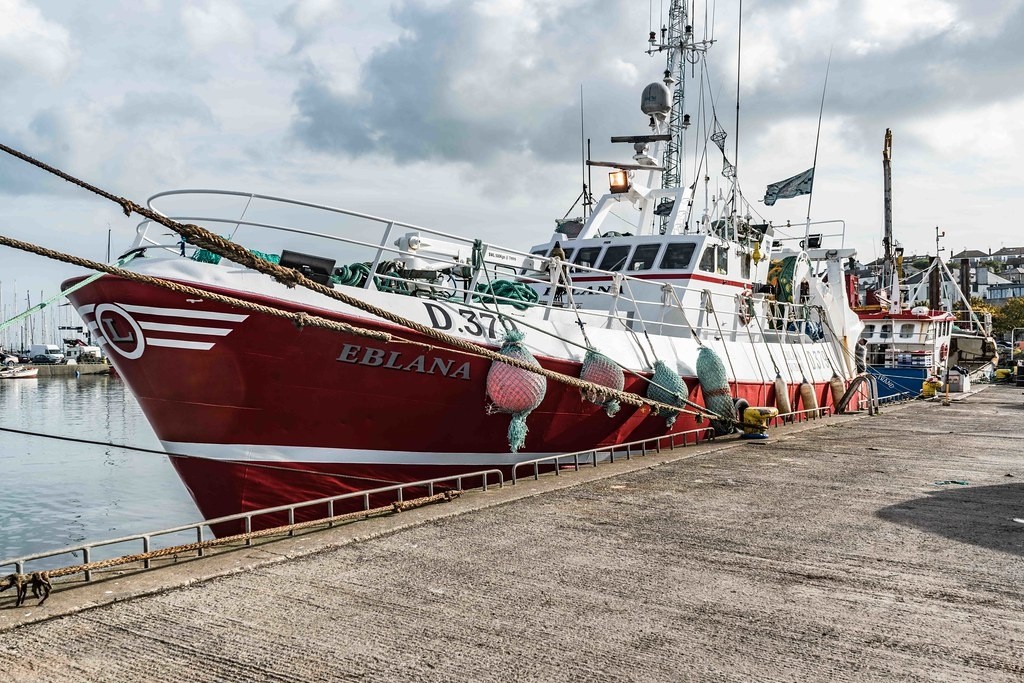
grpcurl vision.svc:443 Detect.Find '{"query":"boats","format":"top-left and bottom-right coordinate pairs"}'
top-left (846, 127), bottom-right (1001, 400)
top-left (59, 0), bottom-right (876, 541)
top-left (110, 366), bottom-right (120, 378)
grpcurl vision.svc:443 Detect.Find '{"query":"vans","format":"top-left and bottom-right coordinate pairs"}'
top-left (1014, 341), bottom-right (1024, 350)
top-left (30, 344), bottom-right (64, 363)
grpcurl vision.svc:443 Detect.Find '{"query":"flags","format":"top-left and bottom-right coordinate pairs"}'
top-left (764, 168), bottom-right (814, 206)
top-left (654, 200), bottom-right (675, 216)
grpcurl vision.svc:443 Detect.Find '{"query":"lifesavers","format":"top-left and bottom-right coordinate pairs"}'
top-left (739, 290), bottom-right (754, 324)
top-left (940, 342), bottom-right (948, 361)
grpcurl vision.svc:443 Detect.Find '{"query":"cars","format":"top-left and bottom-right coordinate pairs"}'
top-left (0, 352), bottom-right (18, 368)
top-left (996, 340), bottom-right (1014, 352)
top-left (9, 352), bottom-right (30, 363)
